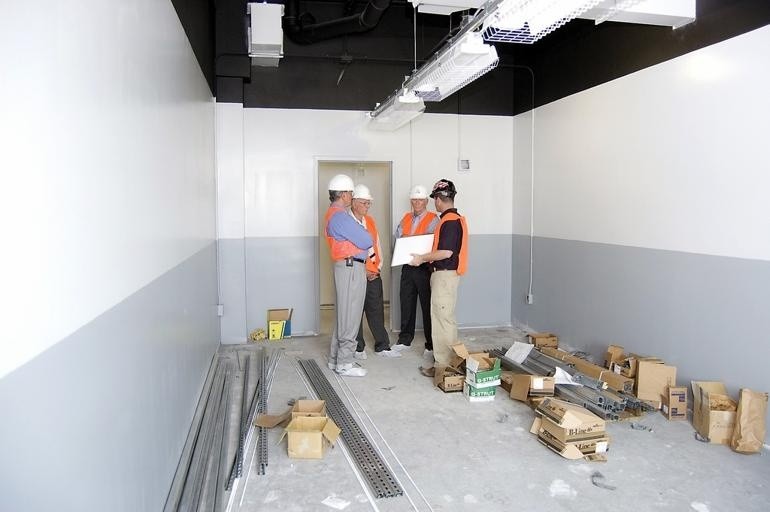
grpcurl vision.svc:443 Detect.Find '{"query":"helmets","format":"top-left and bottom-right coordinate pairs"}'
top-left (409, 184), bottom-right (429, 199)
top-left (430, 180), bottom-right (457, 198)
top-left (352, 184), bottom-right (373, 200)
top-left (328, 174), bottom-right (354, 192)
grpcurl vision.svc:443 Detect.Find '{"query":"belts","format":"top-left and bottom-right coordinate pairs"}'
top-left (429, 267), bottom-right (443, 272)
top-left (353, 258), bottom-right (366, 264)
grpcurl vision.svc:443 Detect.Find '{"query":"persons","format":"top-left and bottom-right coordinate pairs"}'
top-left (324, 174), bottom-right (373, 377)
top-left (391, 187), bottom-right (440, 361)
top-left (347, 184), bottom-right (403, 360)
top-left (410, 180), bottom-right (467, 377)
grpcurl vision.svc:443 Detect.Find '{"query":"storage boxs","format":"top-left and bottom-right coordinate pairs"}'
top-left (266, 307), bottom-right (291, 342)
top-left (276, 416), bottom-right (341, 460)
top-left (690, 378), bottom-right (767, 455)
top-left (531, 399), bottom-right (611, 460)
top-left (433, 329), bottom-right (555, 405)
top-left (252, 399), bottom-right (326, 428)
top-left (544, 342), bottom-right (687, 424)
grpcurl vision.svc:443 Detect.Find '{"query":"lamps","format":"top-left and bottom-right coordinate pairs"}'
top-left (403, 0), bottom-right (500, 102)
top-left (481, 1), bottom-right (606, 45)
top-left (369, 76), bottom-right (426, 132)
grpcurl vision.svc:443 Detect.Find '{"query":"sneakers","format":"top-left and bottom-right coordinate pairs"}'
top-left (390, 343), bottom-right (412, 350)
top-left (328, 360), bottom-right (366, 377)
top-left (422, 349), bottom-right (434, 369)
top-left (377, 349), bottom-right (401, 358)
top-left (420, 366), bottom-right (435, 377)
top-left (355, 351), bottom-right (367, 360)
